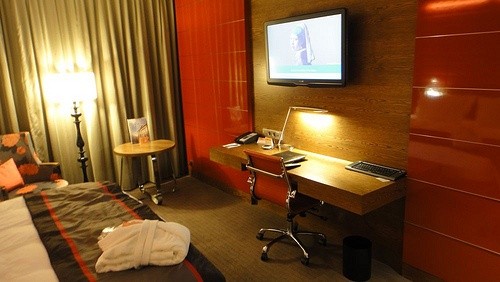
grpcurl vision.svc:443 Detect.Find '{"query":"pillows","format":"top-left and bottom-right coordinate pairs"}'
top-left (0, 157), bottom-right (25, 194)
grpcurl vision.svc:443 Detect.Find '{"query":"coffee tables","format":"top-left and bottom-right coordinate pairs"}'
top-left (113, 140), bottom-right (180, 205)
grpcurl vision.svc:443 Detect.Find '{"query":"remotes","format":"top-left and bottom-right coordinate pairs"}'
top-left (285, 163), bottom-right (301, 169)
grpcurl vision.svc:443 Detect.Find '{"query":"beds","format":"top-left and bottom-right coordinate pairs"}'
top-left (1, 180), bottom-right (228, 282)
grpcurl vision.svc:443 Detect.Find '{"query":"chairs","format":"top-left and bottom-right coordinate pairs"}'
top-left (243, 148), bottom-right (328, 263)
top-left (0, 132), bottom-right (67, 200)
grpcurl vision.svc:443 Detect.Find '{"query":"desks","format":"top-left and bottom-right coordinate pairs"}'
top-left (210, 137), bottom-right (405, 216)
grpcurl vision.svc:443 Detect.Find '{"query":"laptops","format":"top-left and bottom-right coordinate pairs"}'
top-left (271, 151), bottom-right (305, 164)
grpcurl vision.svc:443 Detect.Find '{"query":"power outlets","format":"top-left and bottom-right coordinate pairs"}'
top-left (263, 129), bottom-right (283, 140)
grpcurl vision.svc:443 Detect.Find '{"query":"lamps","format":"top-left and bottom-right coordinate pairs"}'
top-left (410, 77), bottom-right (446, 120)
top-left (276, 107), bottom-right (328, 149)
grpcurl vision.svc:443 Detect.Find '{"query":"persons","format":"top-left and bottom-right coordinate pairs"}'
top-left (290, 23), bottom-right (316, 65)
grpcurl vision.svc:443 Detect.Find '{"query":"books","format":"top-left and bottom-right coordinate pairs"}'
top-left (272, 151), bottom-right (306, 163)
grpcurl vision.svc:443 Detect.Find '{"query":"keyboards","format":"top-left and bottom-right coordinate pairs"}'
top-left (345, 161), bottom-right (407, 181)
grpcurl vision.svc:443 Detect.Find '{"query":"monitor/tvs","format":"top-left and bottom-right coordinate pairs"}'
top-left (264, 7), bottom-right (348, 87)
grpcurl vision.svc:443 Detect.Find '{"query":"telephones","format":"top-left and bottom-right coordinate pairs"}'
top-left (234, 131), bottom-right (259, 145)
